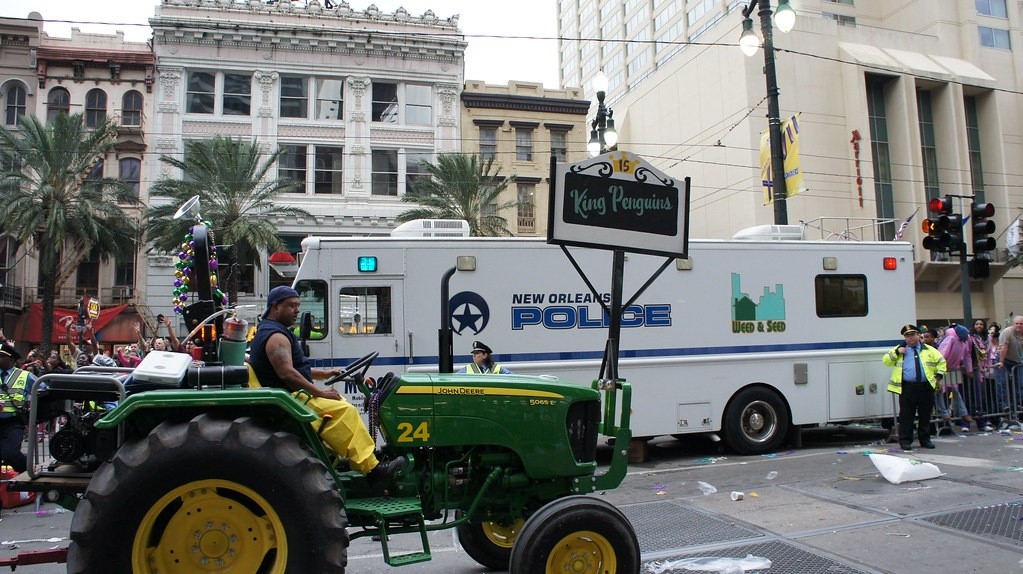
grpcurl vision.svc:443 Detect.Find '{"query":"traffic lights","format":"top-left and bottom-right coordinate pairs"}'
top-left (922, 198), bottom-right (963, 251)
top-left (971, 202), bottom-right (996, 254)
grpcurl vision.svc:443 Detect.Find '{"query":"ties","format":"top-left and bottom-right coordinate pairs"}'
top-left (1, 371), bottom-right (8, 383)
top-left (913, 346), bottom-right (921, 386)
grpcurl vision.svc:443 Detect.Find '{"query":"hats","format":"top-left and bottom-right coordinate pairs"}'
top-left (956, 324), bottom-right (969, 337)
top-left (0, 343), bottom-right (21, 360)
top-left (901, 325), bottom-right (920, 335)
top-left (93, 354), bottom-right (117, 367)
top-left (471, 341), bottom-right (492, 353)
top-left (262, 285), bottom-right (299, 318)
top-left (949, 322), bottom-right (956, 328)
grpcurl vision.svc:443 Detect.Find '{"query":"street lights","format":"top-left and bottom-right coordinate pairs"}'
top-left (740, 0), bottom-right (796, 226)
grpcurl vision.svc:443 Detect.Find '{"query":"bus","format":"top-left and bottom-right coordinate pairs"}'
top-left (288, 218), bottom-right (915, 455)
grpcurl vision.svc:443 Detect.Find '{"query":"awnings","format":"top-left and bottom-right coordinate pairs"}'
top-left (839, 42), bottom-right (997, 89)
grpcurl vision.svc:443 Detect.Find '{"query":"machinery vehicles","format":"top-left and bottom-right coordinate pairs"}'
top-left (0, 150), bottom-right (693, 574)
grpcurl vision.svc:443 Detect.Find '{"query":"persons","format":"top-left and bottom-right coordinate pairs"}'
top-left (310, 315), bottom-right (317, 330)
top-left (917, 315), bottom-right (1023, 438)
top-left (249, 285), bottom-right (405, 485)
top-left (0, 312), bottom-right (196, 475)
top-left (881, 325), bottom-right (947, 451)
top-left (458, 341), bottom-right (513, 375)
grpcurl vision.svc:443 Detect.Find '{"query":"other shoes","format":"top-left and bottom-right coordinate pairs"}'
top-left (944, 416), bottom-right (950, 421)
top-left (980, 426), bottom-right (993, 431)
top-left (961, 427), bottom-right (969, 432)
top-left (366, 456), bottom-right (405, 488)
top-left (963, 415), bottom-right (972, 422)
top-left (901, 443), bottom-right (911, 450)
top-left (921, 440), bottom-right (935, 449)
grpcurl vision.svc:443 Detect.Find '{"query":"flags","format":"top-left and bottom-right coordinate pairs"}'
top-left (893, 210), bottom-right (916, 242)
top-left (936, 216), bottom-right (971, 260)
top-left (1005, 217), bottom-right (1020, 258)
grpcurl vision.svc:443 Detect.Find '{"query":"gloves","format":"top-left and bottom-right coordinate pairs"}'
top-left (935, 373), bottom-right (943, 380)
